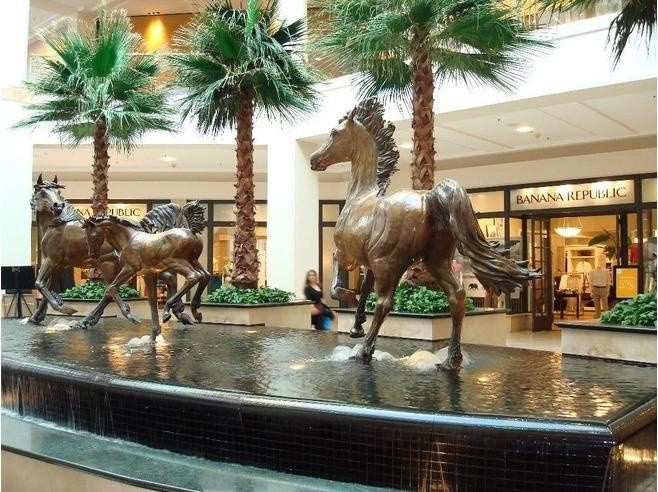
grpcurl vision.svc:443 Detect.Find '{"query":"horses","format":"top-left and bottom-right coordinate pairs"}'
top-left (68, 214), bottom-right (213, 331)
top-left (308, 94), bottom-right (545, 373)
top-left (27, 173), bottom-right (206, 326)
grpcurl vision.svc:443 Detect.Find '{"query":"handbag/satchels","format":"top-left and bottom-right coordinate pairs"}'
top-left (310, 304), bottom-right (325, 315)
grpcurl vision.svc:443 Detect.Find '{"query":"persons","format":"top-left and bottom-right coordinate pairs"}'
top-left (303, 269), bottom-right (329, 330)
top-left (589, 265), bottom-right (610, 319)
